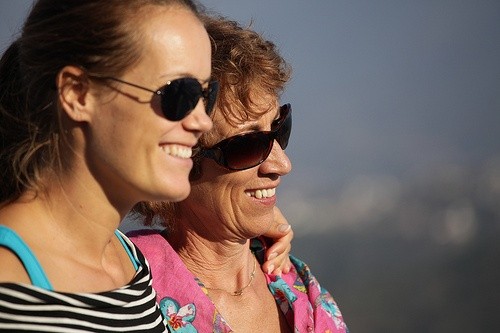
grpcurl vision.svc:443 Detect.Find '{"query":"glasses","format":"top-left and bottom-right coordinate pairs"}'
top-left (95, 76), bottom-right (220, 122)
top-left (201, 103), bottom-right (292, 171)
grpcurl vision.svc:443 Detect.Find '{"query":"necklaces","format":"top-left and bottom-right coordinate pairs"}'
top-left (204, 249), bottom-right (257, 298)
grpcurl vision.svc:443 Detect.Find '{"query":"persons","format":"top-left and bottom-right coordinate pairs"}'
top-left (0, 0), bottom-right (295, 332)
top-left (127, 10), bottom-right (350, 333)
